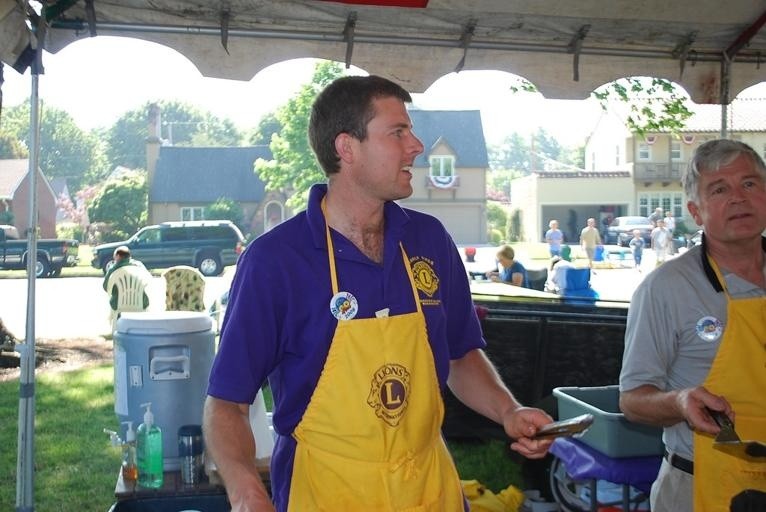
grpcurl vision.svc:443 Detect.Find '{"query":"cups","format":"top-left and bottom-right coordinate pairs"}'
top-left (176, 421), bottom-right (202, 487)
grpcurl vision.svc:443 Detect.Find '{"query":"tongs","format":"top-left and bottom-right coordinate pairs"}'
top-left (505, 411), bottom-right (594, 446)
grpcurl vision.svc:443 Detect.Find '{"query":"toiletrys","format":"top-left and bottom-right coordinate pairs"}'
top-left (136, 402), bottom-right (164, 490)
top-left (118, 421), bottom-right (138, 482)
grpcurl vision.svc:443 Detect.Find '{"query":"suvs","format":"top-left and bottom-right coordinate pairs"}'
top-left (89, 218), bottom-right (249, 279)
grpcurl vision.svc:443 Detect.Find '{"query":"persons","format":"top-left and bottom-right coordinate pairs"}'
top-left (101, 245), bottom-right (150, 332)
top-left (648, 207), bottom-right (664, 225)
top-left (485, 246), bottom-right (529, 287)
top-left (546, 217), bottom-right (566, 263)
top-left (663, 210), bottom-right (680, 256)
top-left (579, 217), bottom-right (602, 274)
top-left (195, 73), bottom-right (559, 511)
top-left (617, 139), bottom-right (766, 511)
top-left (629, 228), bottom-right (646, 272)
top-left (649, 219), bottom-right (674, 266)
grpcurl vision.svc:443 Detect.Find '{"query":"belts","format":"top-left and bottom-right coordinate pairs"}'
top-left (663, 448), bottom-right (693, 474)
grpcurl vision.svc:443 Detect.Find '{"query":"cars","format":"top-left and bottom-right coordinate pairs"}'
top-left (672, 228), bottom-right (706, 249)
top-left (601, 214), bottom-right (654, 246)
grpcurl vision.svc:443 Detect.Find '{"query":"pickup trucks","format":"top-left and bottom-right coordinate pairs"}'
top-left (0, 222), bottom-right (80, 280)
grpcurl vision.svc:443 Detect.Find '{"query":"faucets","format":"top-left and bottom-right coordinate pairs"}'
top-left (102, 428), bottom-right (125, 448)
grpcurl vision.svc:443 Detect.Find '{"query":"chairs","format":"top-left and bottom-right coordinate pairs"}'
top-left (106, 265), bottom-right (206, 331)
top-left (526, 268), bottom-right (548, 291)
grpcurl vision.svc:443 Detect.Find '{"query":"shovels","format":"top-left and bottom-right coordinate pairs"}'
top-left (705, 407), bottom-right (765, 455)
